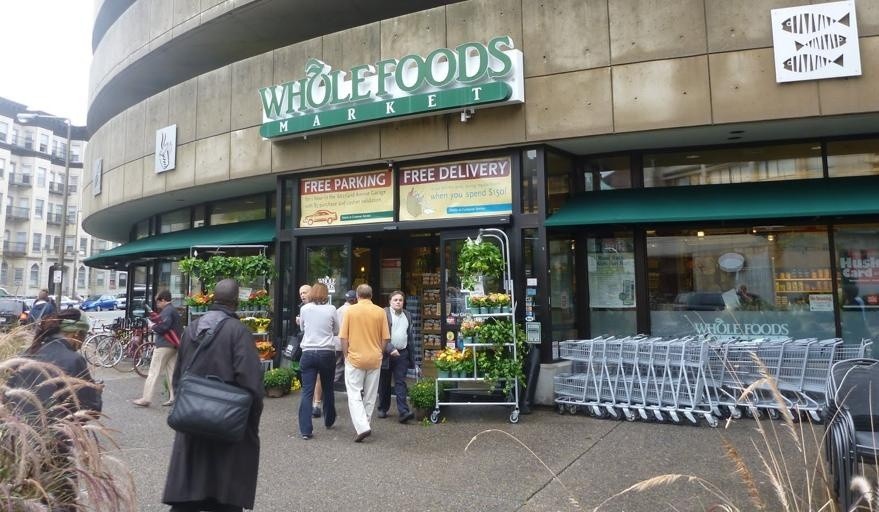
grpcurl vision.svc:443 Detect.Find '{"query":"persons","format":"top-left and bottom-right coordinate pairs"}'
top-left (340, 284), bottom-right (391, 442)
top-left (2, 308), bottom-right (105, 512)
top-left (18, 290), bottom-right (57, 331)
top-left (134, 290), bottom-right (183, 406)
top-left (299, 283), bottom-right (340, 439)
top-left (378, 291), bottom-right (413, 424)
top-left (163, 279), bottom-right (265, 512)
top-left (296, 285), bottom-right (323, 416)
top-left (334, 290), bottom-right (358, 383)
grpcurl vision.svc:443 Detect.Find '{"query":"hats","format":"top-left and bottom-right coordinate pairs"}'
top-left (345, 289), bottom-right (356, 297)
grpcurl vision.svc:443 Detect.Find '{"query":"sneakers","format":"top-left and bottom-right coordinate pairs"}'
top-left (303, 434), bottom-right (313, 439)
top-left (377, 409), bottom-right (387, 417)
top-left (352, 431), bottom-right (371, 442)
top-left (162, 400), bottom-right (176, 406)
top-left (133, 397), bottom-right (151, 406)
top-left (400, 412), bottom-right (414, 423)
top-left (311, 405), bottom-right (322, 417)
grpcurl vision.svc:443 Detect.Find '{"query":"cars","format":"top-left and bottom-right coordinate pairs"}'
top-left (0, 300), bottom-right (29, 327)
top-left (0, 288), bottom-right (23, 300)
top-left (116, 292), bottom-right (126, 302)
top-left (48, 296), bottom-right (78, 309)
top-left (79, 295), bottom-right (119, 311)
top-left (25, 299), bottom-right (37, 308)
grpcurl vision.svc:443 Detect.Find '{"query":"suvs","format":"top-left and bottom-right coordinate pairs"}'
top-left (676, 292), bottom-right (772, 310)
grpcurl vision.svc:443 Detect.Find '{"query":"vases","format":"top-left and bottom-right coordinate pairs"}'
top-left (250, 303), bottom-right (258, 312)
top-left (257, 352), bottom-right (269, 363)
top-left (260, 303), bottom-right (267, 311)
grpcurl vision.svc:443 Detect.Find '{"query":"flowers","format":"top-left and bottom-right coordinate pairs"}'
top-left (255, 342), bottom-right (273, 352)
top-left (247, 293), bottom-right (259, 305)
top-left (256, 290), bottom-right (270, 305)
top-left (185, 294), bottom-right (213, 313)
top-left (240, 316), bottom-right (271, 334)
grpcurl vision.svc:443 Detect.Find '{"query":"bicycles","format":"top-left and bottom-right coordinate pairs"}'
top-left (81, 317), bottom-right (156, 377)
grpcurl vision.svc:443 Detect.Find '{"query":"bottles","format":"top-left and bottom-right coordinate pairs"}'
top-left (146, 317), bottom-right (156, 334)
top-left (791, 265), bottom-right (811, 279)
top-left (560, 288), bottom-right (571, 310)
top-left (405, 294), bottom-right (423, 379)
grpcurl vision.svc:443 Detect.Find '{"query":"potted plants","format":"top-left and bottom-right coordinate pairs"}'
top-left (264, 368), bottom-right (296, 398)
top-left (240, 301), bottom-right (246, 311)
top-left (432, 239), bottom-right (532, 401)
top-left (408, 377), bottom-right (444, 420)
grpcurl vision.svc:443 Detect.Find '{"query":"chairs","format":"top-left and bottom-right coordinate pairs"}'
top-left (822, 358), bottom-right (879, 512)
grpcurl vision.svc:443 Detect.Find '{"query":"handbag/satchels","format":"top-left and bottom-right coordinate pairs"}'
top-left (168, 373), bottom-right (254, 444)
top-left (284, 335), bottom-right (301, 361)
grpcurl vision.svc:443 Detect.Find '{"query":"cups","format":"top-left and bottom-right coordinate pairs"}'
top-left (622, 280), bottom-right (636, 306)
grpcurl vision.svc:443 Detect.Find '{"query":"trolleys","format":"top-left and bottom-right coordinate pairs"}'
top-left (431, 229), bottom-right (521, 426)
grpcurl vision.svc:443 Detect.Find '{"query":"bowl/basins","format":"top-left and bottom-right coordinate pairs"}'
top-left (718, 250), bottom-right (747, 273)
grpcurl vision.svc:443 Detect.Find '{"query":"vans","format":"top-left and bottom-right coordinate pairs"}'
top-left (116, 284), bottom-right (153, 310)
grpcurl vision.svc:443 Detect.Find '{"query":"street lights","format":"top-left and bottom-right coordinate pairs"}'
top-left (16, 110), bottom-right (73, 306)
